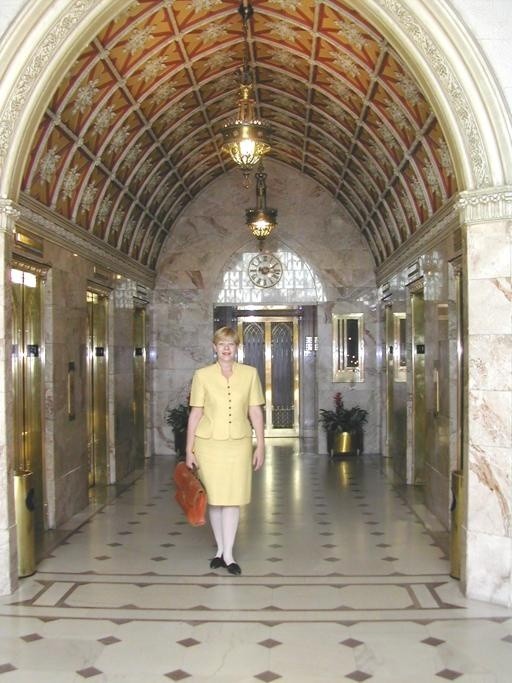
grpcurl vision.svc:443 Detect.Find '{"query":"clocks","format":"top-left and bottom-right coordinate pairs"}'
top-left (248, 251), bottom-right (283, 288)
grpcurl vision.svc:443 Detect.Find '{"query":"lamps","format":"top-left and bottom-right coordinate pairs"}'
top-left (217, 1), bottom-right (275, 189)
top-left (244, 159), bottom-right (278, 254)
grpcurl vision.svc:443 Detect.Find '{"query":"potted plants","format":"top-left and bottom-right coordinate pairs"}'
top-left (317, 390), bottom-right (369, 457)
top-left (166, 393), bottom-right (191, 459)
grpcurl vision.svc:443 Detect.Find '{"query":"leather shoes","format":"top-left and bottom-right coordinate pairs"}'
top-left (223, 562), bottom-right (243, 576)
top-left (208, 552), bottom-right (223, 569)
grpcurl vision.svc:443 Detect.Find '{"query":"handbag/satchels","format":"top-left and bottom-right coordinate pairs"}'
top-left (173, 458), bottom-right (209, 528)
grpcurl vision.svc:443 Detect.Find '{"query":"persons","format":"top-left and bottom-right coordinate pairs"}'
top-left (183, 325), bottom-right (267, 575)
